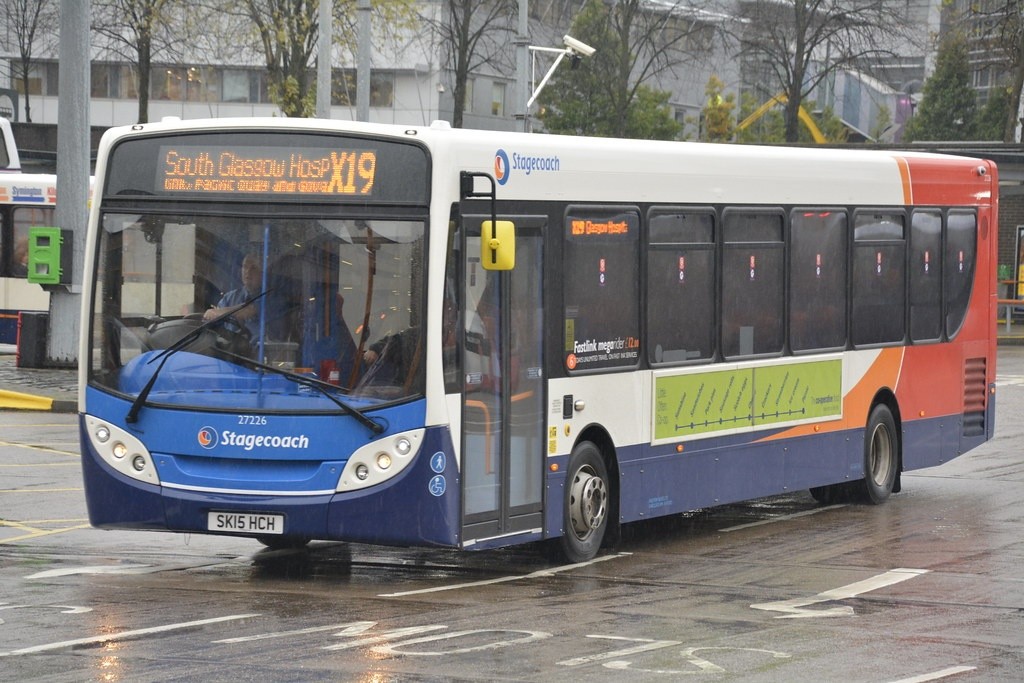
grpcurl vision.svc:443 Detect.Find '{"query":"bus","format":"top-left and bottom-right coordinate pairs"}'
top-left (0, 116), bottom-right (199, 344)
top-left (74, 123), bottom-right (1001, 566)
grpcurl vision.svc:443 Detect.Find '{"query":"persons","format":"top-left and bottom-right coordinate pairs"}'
top-left (15, 236), bottom-right (28, 276)
top-left (204, 252), bottom-right (284, 347)
top-left (364, 322), bottom-right (422, 387)
top-left (444, 309), bottom-right (502, 390)
